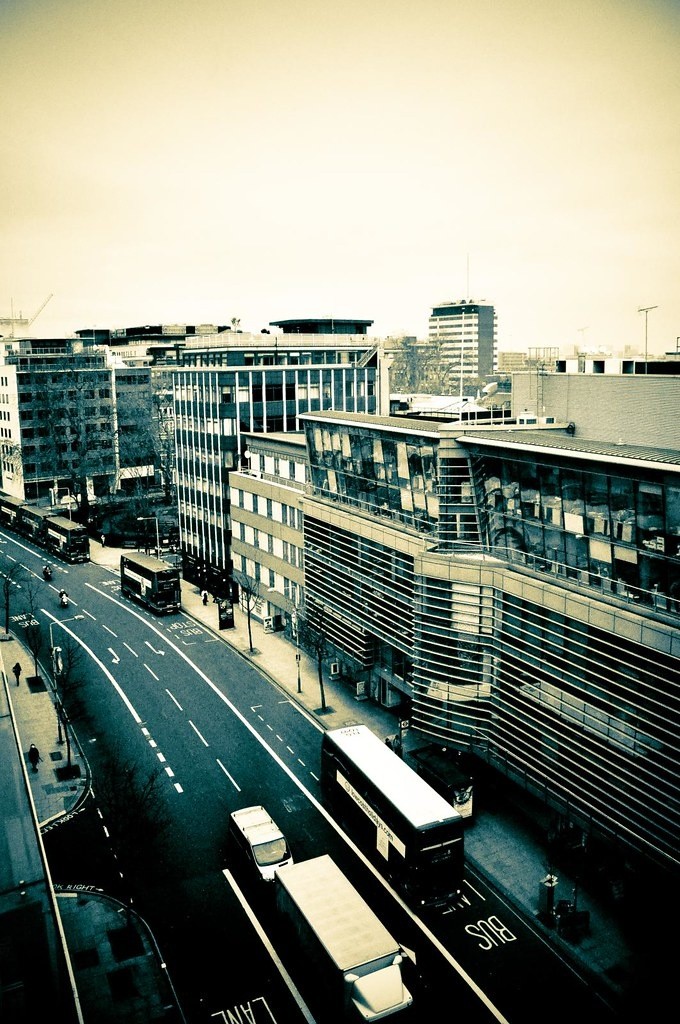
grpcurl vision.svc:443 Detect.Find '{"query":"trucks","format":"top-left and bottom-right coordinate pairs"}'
top-left (274, 854), bottom-right (416, 1023)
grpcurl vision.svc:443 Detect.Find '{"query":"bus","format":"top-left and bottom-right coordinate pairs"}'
top-left (120, 552), bottom-right (182, 617)
top-left (322, 724), bottom-right (467, 914)
top-left (0, 495), bottom-right (90, 565)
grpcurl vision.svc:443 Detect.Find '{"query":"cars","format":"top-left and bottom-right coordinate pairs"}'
top-left (231, 805), bottom-right (294, 883)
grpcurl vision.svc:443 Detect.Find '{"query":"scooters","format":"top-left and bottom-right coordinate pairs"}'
top-left (62, 594), bottom-right (68, 607)
top-left (43, 569), bottom-right (52, 580)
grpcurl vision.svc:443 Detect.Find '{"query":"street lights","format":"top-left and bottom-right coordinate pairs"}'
top-left (52, 488), bottom-right (72, 521)
top-left (50, 615), bottom-right (86, 697)
top-left (137, 516), bottom-right (161, 560)
top-left (267, 586), bottom-right (303, 693)
